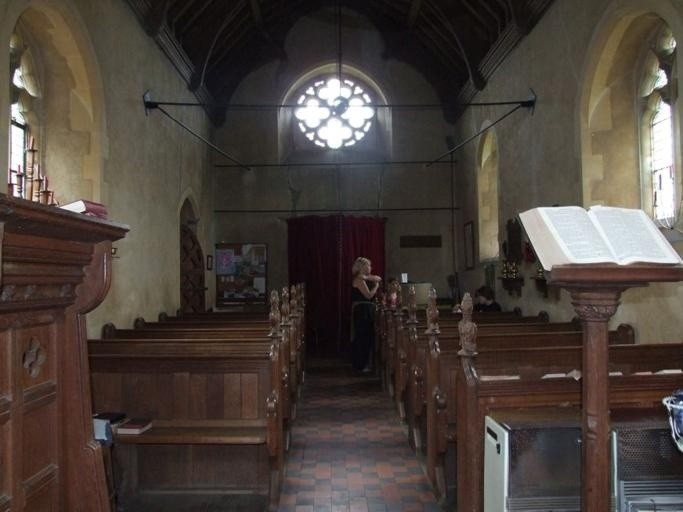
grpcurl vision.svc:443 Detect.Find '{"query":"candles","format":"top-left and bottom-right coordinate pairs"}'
top-left (43, 176), bottom-right (47, 191)
top-left (17, 165), bottom-right (20, 172)
top-left (36, 164), bottom-right (40, 179)
top-left (29, 135), bottom-right (35, 149)
top-left (9, 168), bottom-right (12, 182)
top-left (50, 191), bottom-right (55, 204)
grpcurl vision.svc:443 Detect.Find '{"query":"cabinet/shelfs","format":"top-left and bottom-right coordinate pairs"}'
top-left (0, 192), bottom-right (131, 509)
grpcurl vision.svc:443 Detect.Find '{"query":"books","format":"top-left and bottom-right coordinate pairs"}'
top-left (91, 411), bottom-right (153, 441)
top-left (58, 199), bottom-right (108, 219)
top-left (519, 205), bottom-right (682, 273)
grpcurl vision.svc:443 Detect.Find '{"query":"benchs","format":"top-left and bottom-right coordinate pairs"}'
top-left (88, 281), bottom-right (306, 511)
top-left (378, 281), bottom-right (682, 512)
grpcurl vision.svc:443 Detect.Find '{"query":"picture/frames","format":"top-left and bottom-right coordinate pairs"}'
top-left (463, 220), bottom-right (475, 271)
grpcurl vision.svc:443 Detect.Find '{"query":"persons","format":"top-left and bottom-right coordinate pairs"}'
top-left (452, 286), bottom-right (502, 313)
top-left (349, 256), bottom-right (382, 374)
